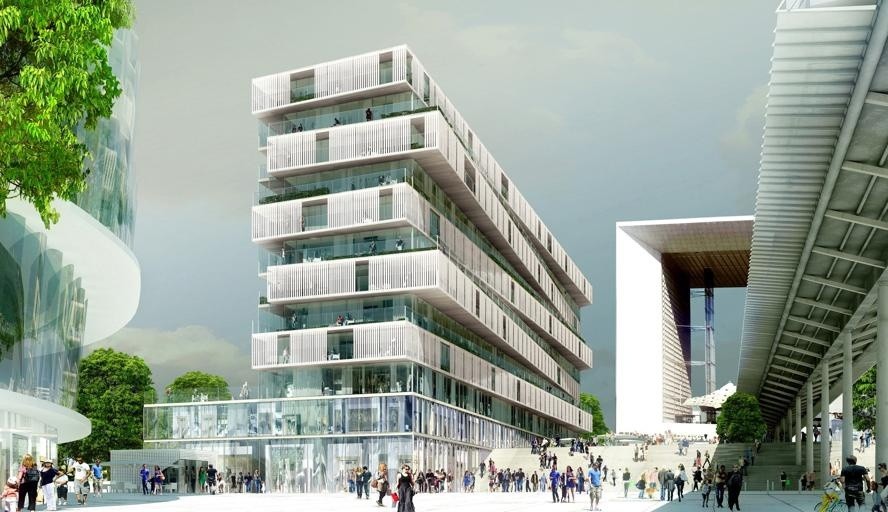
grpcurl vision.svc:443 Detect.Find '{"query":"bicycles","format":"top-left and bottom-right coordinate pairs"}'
top-left (813, 487), bottom-right (849, 512)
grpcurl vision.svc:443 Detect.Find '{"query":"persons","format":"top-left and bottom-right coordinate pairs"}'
top-left (396, 236), bottom-right (404, 250)
top-left (329, 347), bottom-right (337, 360)
top-left (369, 240), bottom-right (377, 255)
top-left (280, 247), bottom-right (288, 264)
top-left (345, 312), bottom-right (353, 325)
top-left (292, 314), bottom-right (297, 329)
top-left (282, 346), bottom-right (289, 363)
top-left (338, 315), bottom-right (344, 325)
top-left (302, 216), bottom-right (306, 231)
top-left (298, 123), bottom-right (303, 132)
top-left (333, 118), bottom-right (340, 125)
top-left (365, 107), bottom-right (373, 121)
top-left (378, 174), bottom-right (387, 185)
